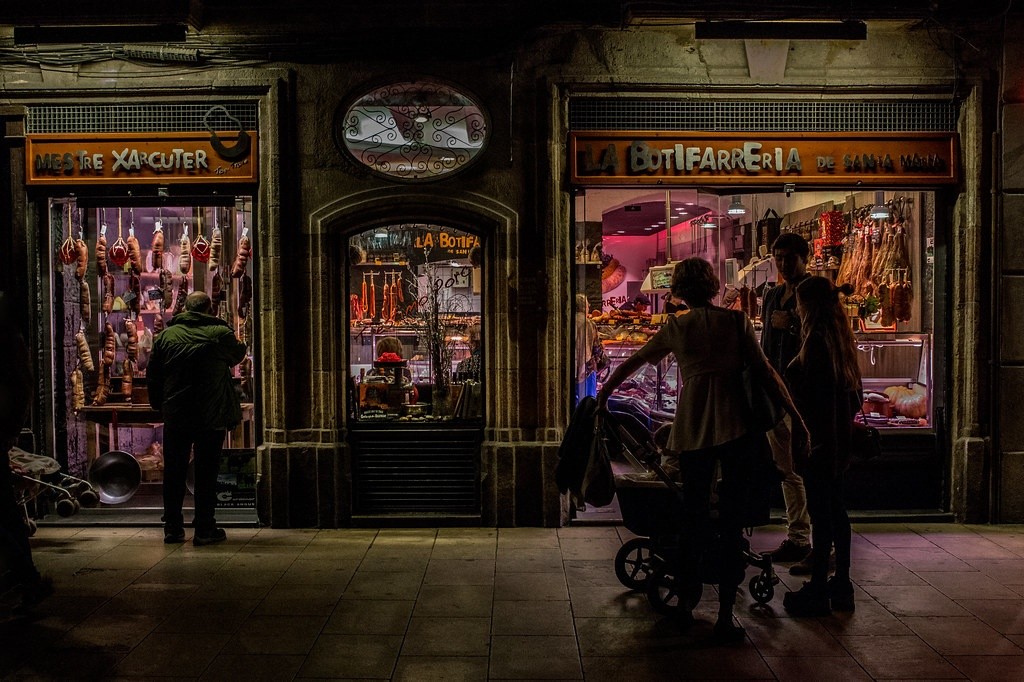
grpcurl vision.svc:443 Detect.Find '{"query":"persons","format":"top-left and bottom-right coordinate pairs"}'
top-left (759, 232), bottom-right (836, 577)
top-left (618, 295), bottom-right (651, 324)
top-left (456, 325), bottom-right (481, 381)
top-left (663, 295), bottom-right (688, 314)
top-left (783, 276), bottom-right (863, 615)
top-left (377, 337), bottom-right (418, 404)
top-left (574, 294), bottom-right (601, 406)
top-left (147, 291), bottom-right (247, 546)
top-left (596, 257), bottom-right (811, 639)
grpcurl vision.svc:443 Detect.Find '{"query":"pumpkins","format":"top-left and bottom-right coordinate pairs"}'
top-left (882, 385), bottom-right (926, 418)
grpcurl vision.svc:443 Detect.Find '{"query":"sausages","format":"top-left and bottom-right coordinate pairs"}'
top-left (602, 258), bottom-right (626, 294)
top-left (876, 279), bottom-right (912, 326)
top-left (73, 233), bottom-right (249, 405)
top-left (589, 310), bottom-right (652, 322)
top-left (362, 278), bottom-right (405, 322)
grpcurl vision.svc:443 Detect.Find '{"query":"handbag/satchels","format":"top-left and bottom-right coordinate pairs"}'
top-left (582, 411), bottom-right (615, 507)
top-left (851, 419), bottom-right (885, 509)
top-left (735, 311), bottom-right (784, 431)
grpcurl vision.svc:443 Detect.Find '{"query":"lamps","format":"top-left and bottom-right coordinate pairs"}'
top-left (728, 195), bottom-right (745, 215)
top-left (870, 192), bottom-right (889, 219)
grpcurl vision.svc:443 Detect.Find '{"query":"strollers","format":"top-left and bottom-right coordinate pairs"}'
top-left (9, 445), bottom-right (100, 536)
top-left (586, 396), bottom-right (779, 606)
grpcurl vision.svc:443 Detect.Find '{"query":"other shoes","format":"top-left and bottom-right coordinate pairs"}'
top-left (193, 526), bottom-right (224, 545)
top-left (785, 574), bottom-right (828, 614)
top-left (165, 529), bottom-right (185, 542)
top-left (759, 540), bottom-right (812, 561)
top-left (714, 621), bottom-right (744, 641)
top-left (677, 583), bottom-right (703, 613)
top-left (789, 547), bottom-right (832, 573)
top-left (827, 574), bottom-right (856, 611)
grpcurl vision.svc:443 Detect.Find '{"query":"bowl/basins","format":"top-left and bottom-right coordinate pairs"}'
top-left (89, 450), bottom-right (143, 504)
